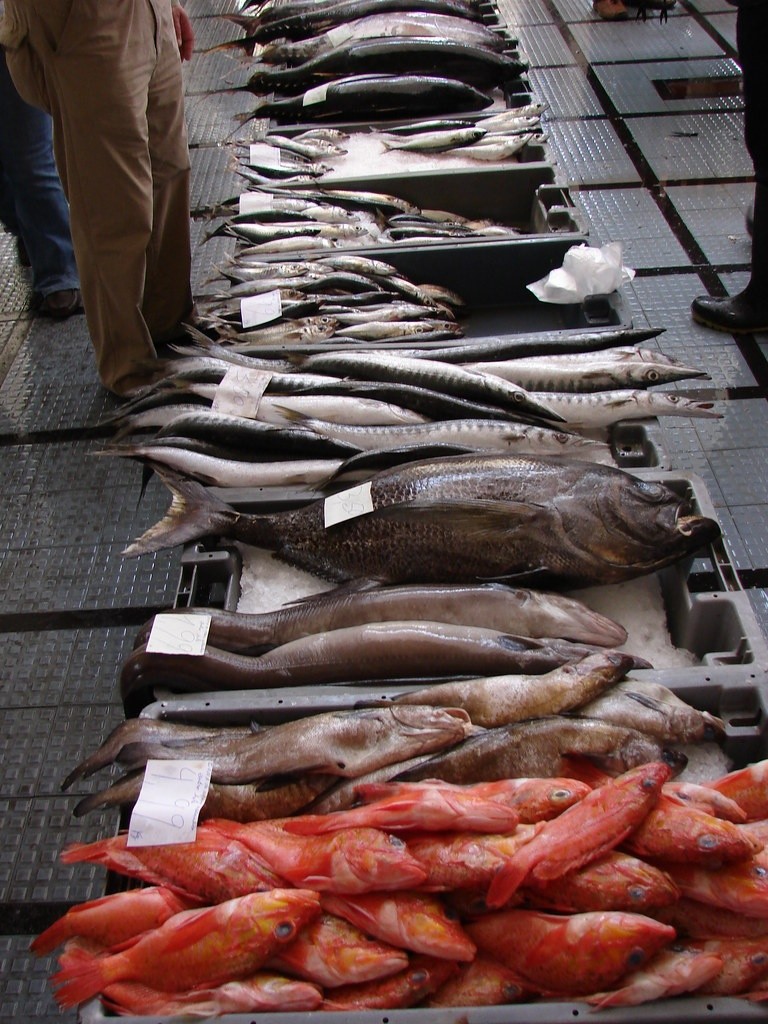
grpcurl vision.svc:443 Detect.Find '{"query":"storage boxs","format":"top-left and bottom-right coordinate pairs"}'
top-left (78, 0), bottom-right (768, 1024)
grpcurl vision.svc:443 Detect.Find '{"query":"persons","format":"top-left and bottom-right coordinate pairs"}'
top-left (691, 0), bottom-right (768, 333)
top-left (0, 0), bottom-right (219, 403)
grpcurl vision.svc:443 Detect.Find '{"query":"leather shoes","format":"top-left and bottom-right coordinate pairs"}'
top-left (45, 288), bottom-right (81, 319)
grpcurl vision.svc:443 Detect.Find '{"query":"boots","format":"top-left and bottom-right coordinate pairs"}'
top-left (690, 180), bottom-right (768, 334)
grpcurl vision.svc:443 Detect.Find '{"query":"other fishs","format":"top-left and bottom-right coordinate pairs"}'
top-left (30, 0), bottom-right (768, 1019)
